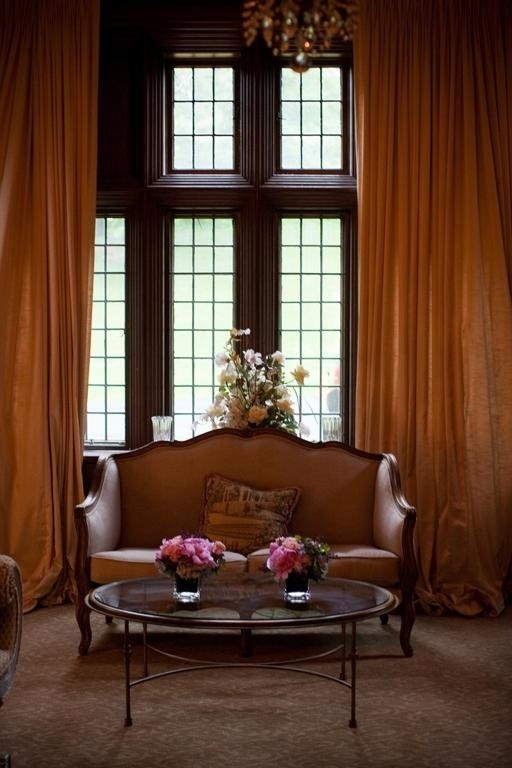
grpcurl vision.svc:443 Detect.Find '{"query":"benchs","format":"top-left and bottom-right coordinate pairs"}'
top-left (73, 426), bottom-right (419, 668)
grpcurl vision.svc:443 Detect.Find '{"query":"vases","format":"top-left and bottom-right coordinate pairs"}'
top-left (172, 572), bottom-right (200, 603)
top-left (282, 571), bottom-right (313, 602)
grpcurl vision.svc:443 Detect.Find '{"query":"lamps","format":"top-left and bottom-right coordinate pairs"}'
top-left (240, 2), bottom-right (362, 66)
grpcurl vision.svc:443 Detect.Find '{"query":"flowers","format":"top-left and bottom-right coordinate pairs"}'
top-left (265, 534), bottom-right (336, 583)
top-left (192, 328), bottom-right (312, 440)
top-left (152, 535), bottom-right (227, 581)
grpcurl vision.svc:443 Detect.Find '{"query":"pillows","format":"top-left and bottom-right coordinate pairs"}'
top-left (190, 470), bottom-right (301, 555)
